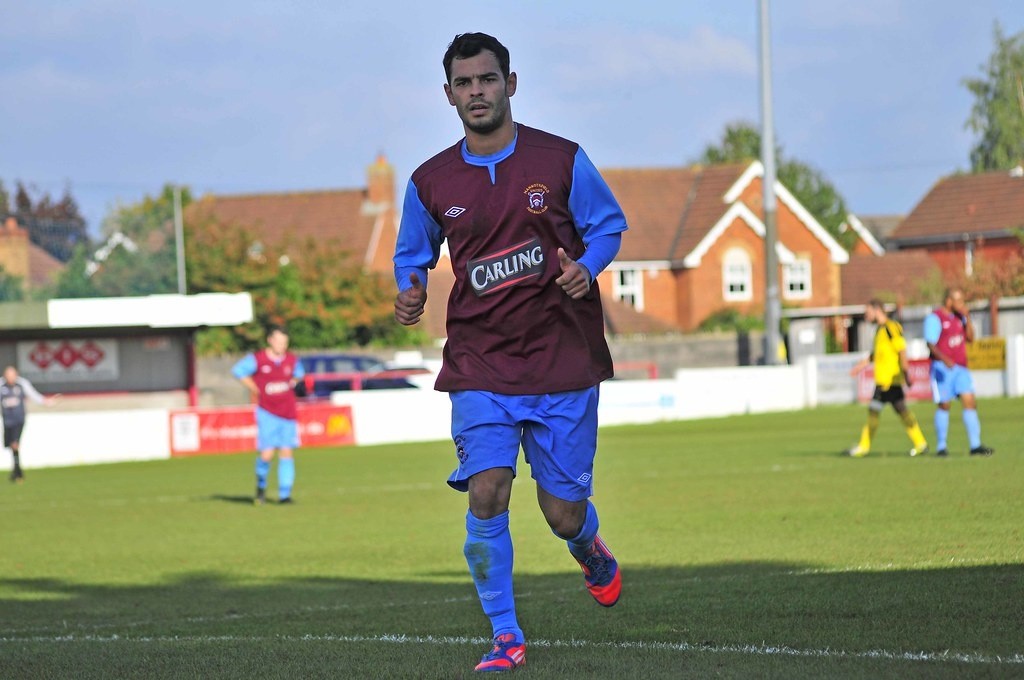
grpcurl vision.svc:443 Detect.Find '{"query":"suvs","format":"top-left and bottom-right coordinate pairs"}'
top-left (288, 354), bottom-right (418, 397)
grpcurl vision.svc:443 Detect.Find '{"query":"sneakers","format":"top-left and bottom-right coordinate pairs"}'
top-left (475, 633), bottom-right (526, 672)
top-left (568, 533), bottom-right (621, 607)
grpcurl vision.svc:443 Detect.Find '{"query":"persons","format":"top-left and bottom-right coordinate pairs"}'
top-left (232, 324), bottom-right (306, 504)
top-left (393, 32), bottom-right (629, 670)
top-left (848, 300), bottom-right (930, 457)
top-left (924, 287), bottom-right (995, 457)
top-left (0, 365), bottom-right (61, 482)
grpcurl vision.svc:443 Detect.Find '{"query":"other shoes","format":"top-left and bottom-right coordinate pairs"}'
top-left (255, 491), bottom-right (264, 505)
top-left (970, 446), bottom-right (994, 456)
top-left (279, 498), bottom-right (291, 504)
top-left (910, 445), bottom-right (929, 455)
top-left (11, 471), bottom-right (22, 482)
top-left (938, 449), bottom-right (946, 455)
top-left (847, 447), bottom-right (868, 457)
